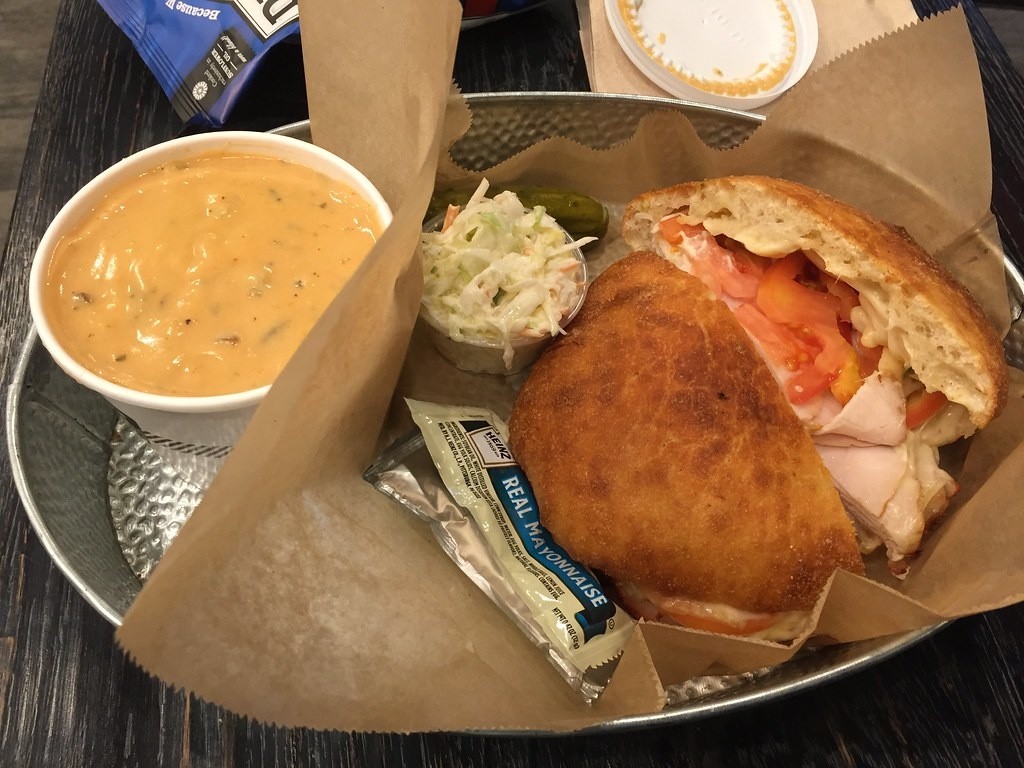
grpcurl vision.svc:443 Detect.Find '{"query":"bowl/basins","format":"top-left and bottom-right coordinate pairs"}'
top-left (27, 132), bottom-right (392, 446)
top-left (410, 202), bottom-right (590, 379)
top-left (6, 90), bottom-right (1024, 738)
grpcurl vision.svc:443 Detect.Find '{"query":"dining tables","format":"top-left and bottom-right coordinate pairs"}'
top-left (1, 0), bottom-right (1024, 768)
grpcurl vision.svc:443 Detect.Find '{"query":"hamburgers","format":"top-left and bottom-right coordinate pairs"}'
top-left (508, 174), bottom-right (1012, 641)
